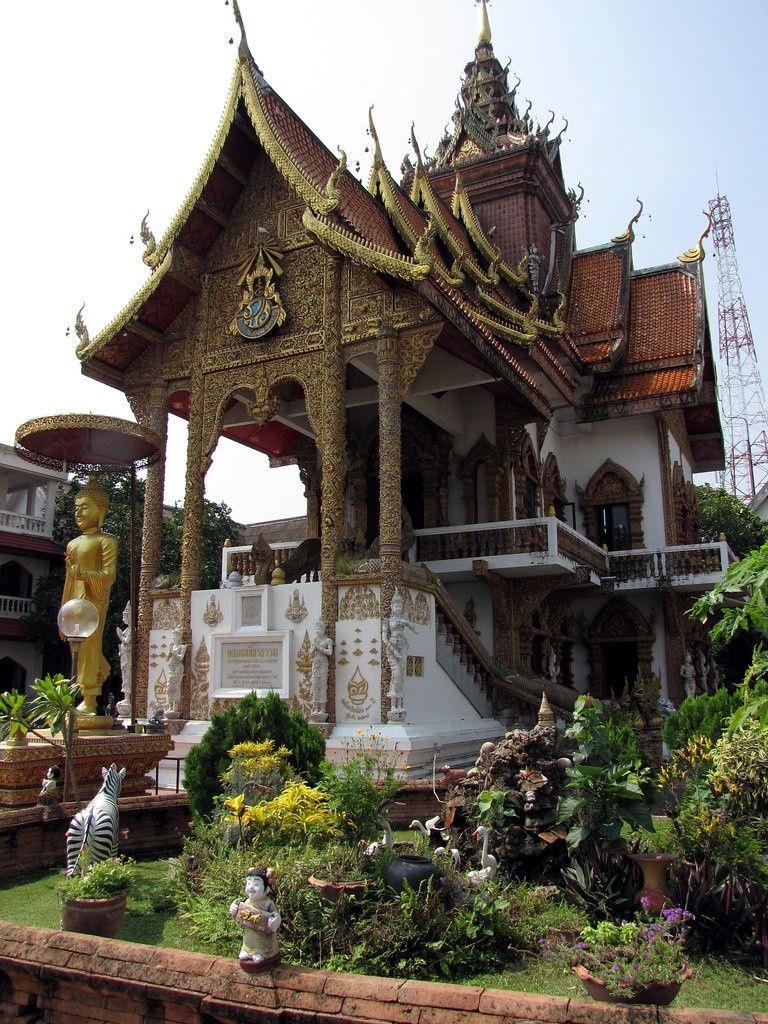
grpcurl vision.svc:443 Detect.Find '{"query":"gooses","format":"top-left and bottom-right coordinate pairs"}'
top-left (358, 798), bottom-right (463, 859)
top-left (472, 826), bottom-right (498, 873)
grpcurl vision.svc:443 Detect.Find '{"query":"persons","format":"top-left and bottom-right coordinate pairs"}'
top-left (57, 469), bottom-right (119, 732)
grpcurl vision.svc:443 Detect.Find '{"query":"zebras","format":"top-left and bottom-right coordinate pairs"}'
top-left (65, 762), bottom-right (127, 878)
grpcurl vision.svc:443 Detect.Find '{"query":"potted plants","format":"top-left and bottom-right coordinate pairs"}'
top-left (542, 898), bottom-right (589, 948)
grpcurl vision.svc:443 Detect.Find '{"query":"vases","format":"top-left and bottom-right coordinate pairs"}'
top-left (571, 957), bottom-right (692, 1005)
top-left (308, 873), bottom-right (375, 903)
top-left (384, 852), bottom-right (442, 898)
top-left (58, 891), bottom-right (128, 937)
top-left (630, 852), bottom-right (677, 916)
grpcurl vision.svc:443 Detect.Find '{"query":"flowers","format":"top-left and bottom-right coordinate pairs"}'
top-left (54, 854), bottom-right (141, 902)
top-left (319, 724), bottom-right (412, 883)
top-left (540, 897), bottom-right (694, 998)
